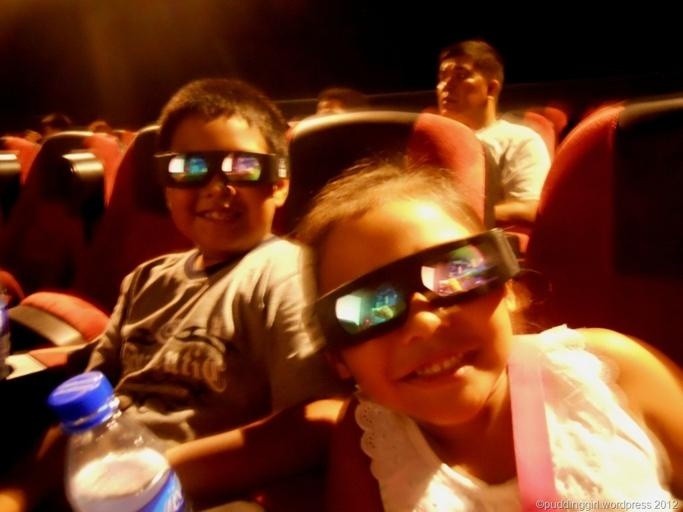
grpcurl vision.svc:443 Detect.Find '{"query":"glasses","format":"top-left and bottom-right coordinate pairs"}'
top-left (313, 227), bottom-right (521, 352)
top-left (154, 150), bottom-right (288, 188)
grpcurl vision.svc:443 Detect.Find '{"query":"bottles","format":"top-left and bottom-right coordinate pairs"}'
top-left (42, 368), bottom-right (195, 512)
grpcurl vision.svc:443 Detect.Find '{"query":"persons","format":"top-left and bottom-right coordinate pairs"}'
top-left (314, 87), bottom-right (370, 117)
top-left (435, 38), bottom-right (553, 225)
top-left (295, 153), bottom-right (682, 512)
top-left (0, 75), bottom-right (328, 512)
top-left (23, 113), bottom-right (72, 145)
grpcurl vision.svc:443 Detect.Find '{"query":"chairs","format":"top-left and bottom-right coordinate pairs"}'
top-left (0, 98), bottom-right (683, 512)
